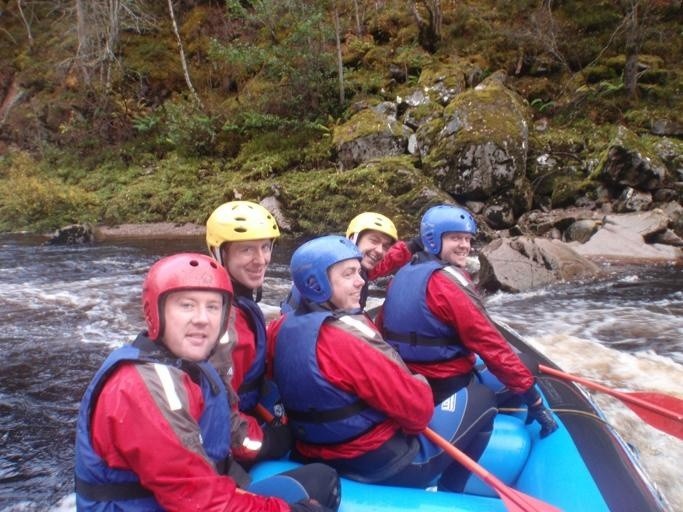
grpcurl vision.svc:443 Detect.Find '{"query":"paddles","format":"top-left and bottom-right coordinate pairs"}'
top-left (538, 365), bottom-right (683, 440)
top-left (424, 428), bottom-right (561, 512)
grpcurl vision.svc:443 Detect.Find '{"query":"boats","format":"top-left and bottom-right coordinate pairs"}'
top-left (245, 302), bottom-right (668, 511)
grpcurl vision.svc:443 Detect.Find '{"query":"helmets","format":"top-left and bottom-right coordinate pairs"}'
top-left (206, 201), bottom-right (280, 259)
top-left (290, 235), bottom-right (363, 304)
top-left (420, 204), bottom-right (476, 255)
top-left (142, 253), bottom-right (234, 340)
top-left (345, 212), bottom-right (398, 245)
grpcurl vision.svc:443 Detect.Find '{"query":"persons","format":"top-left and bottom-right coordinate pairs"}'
top-left (375, 204), bottom-right (559, 440)
top-left (205, 200), bottom-right (293, 476)
top-left (278, 212), bottom-right (423, 315)
top-left (266, 235), bottom-right (499, 494)
top-left (74, 253), bottom-right (342, 512)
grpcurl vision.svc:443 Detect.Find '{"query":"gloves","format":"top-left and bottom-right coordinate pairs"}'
top-left (292, 499), bottom-right (332, 512)
top-left (522, 388), bottom-right (558, 436)
top-left (259, 423), bottom-right (291, 460)
